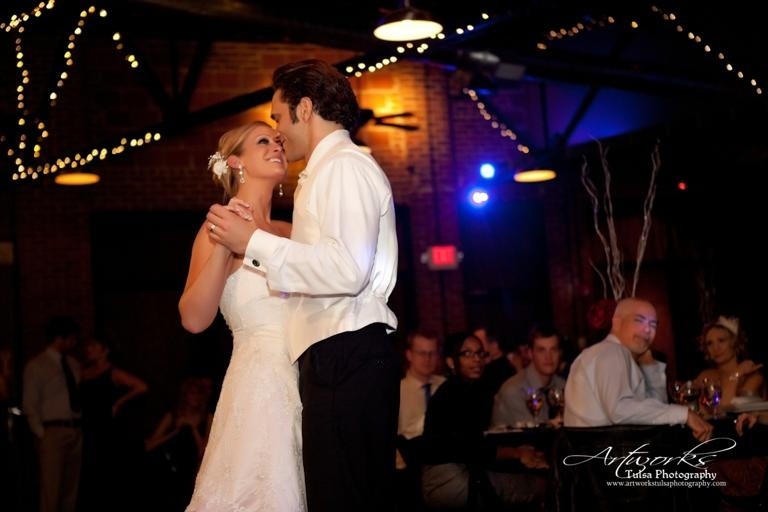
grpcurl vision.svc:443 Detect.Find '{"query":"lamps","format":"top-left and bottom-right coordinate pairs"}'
top-left (370, 0), bottom-right (445, 42)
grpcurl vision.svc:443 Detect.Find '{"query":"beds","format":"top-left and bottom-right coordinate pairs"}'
top-left (484, 412), bottom-right (768, 512)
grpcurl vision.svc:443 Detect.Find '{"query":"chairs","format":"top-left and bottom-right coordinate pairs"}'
top-left (559, 425), bottom-right (670, 512)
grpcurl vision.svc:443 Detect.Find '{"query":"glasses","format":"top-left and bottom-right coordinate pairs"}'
top-left (460, 350), bottom-right (490, 358)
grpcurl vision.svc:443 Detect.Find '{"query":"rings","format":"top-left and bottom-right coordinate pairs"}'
top-left (209, 223), bottom-right (216, 234)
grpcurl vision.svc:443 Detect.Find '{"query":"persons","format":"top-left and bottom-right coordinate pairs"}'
top-left (177, 118), bottom-right (310, 512)
top-left (206, 58), bottom-right (407, 512)
top-left (0, 294), bottom-right (768, 511)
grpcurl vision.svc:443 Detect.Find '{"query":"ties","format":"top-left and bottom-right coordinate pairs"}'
top-left (423, 383), bottom-right (432, 403)
top-left (61, 356), bottom-right (81, 412)
top-left (541, 387), bottom-right (560, 420)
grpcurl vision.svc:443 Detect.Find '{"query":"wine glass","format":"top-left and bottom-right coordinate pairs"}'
top-left (728, 368), bottom-right (744, 400)
top-left (524, 384), bottom-right (564, 428)
top-left (675, 378), bottom-right (721, 422)
